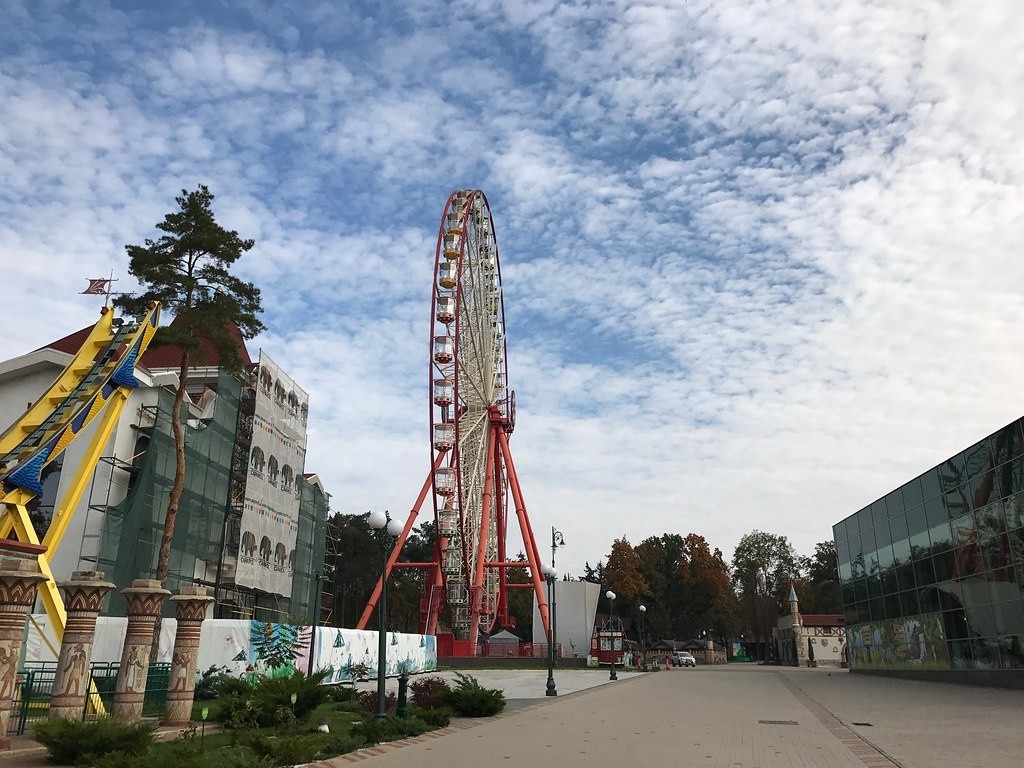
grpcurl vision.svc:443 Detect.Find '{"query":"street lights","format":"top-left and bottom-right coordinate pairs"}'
top-left (550, 525), bottom-right (568, 669)
top-left (606, 590), bottom-right (617, 680)
top-left (368, 511), bottom-right (404, 723)
top-left (698, 630), bottom-right (709, 664)
top-left (639, 605), bottom-right (649, 672)
top-left (540, 562), bottom-right (558, 697)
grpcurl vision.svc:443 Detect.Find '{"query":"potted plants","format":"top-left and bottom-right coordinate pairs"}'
top-left (806, 637), bottom-right (815, 668)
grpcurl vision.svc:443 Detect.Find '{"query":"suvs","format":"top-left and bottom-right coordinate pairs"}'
top-left (672, 651), bottom-right (696, 667)
